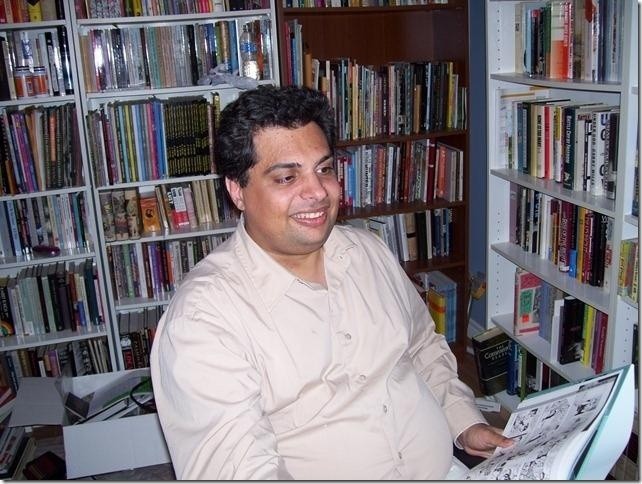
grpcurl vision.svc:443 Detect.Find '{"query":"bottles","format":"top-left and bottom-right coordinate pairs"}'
top-left (13, 66), bottom-right (36, 99)
top-left (32, 66), bottom-right (50, 98)
top-left (239, 24), bottom-right (261, 80)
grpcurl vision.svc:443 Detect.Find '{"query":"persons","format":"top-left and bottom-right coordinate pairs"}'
top-left (150, 81), bottom-right (515, 482)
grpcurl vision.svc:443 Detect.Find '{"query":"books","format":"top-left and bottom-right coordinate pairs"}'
top-left (0, 1), bottom-right (271, 483)
top-left (514, 1), bottom-right (629, 85)
top-left (466, 363), bottom-right (635, 480)
top-left (509, 181), bottom-right (640, 408)
top-left (495, 87), bottom-right (640, 219)
top-left (279, 0), bottom-right (467, 344)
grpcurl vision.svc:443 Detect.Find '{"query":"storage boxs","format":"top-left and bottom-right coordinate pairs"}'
top-left (8, 368), bottom-right (181, 479)
